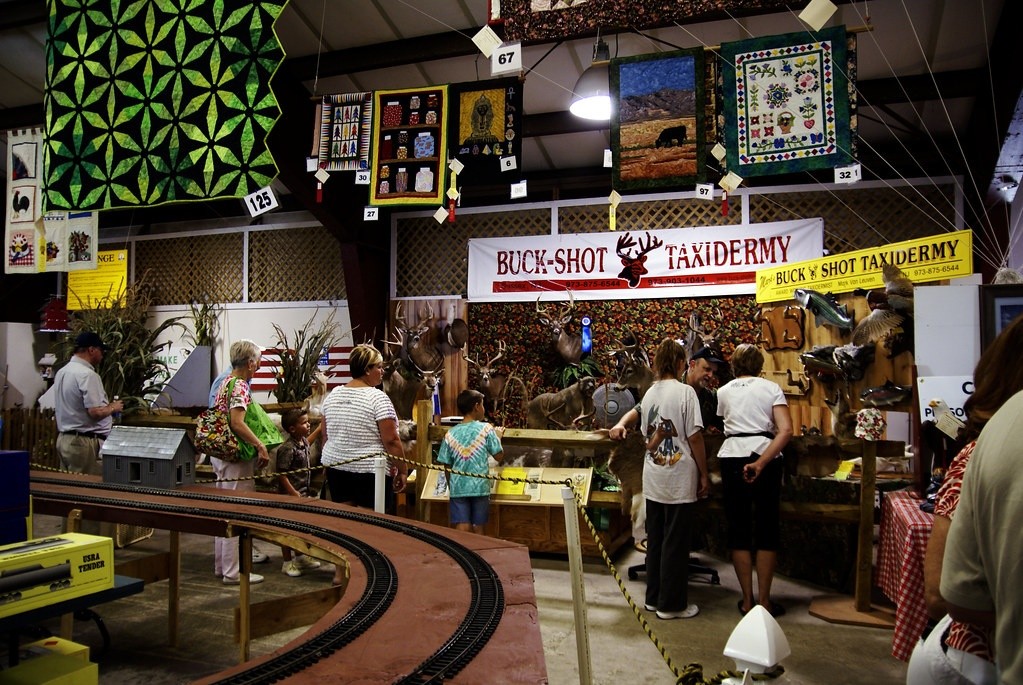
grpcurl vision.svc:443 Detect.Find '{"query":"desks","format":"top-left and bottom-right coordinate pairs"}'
top-left (878, 483), bottom-right (944, 660)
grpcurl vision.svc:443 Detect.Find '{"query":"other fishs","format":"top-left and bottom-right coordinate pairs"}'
top-left (798, 344), bottom-right (913, 442)
top-left (794, 288), bottom-right (856, 332)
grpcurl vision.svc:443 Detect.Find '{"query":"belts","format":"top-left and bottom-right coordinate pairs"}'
top-left (63, 429), bottom-right (107, 441)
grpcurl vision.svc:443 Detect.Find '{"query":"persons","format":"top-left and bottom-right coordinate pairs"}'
top-left (54, 332), bottom-right (123, 475)
top-left (275, 409), bottom-right (321, 577)
top-left (318, 343), bottom-right (409, 587)
top-left (716, 344), bottom-right (794, 619)
top-left (640, 339), bottom-right (710, 620)
top-left (436, 390), bottom-right (506, 536)
top-left (923, 317), bottom-right (1022, 685)
top-left (608, 345), bottom-right (724, 441)
top-left (208, 342), bottom-right (269, 584)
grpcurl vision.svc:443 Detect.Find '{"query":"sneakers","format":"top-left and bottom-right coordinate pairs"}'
top-left (644, 600), bottom-right (660, 612)
top-left (222, 571), bottom-right (264, 584)
top-left (251, 545), bottom-right (267, 562)
top-left (295, 555), bottom-right (321, 568)
top-left (281, 561), bottom-right (300, 577)
top-left (656, 605), bottom-right (701, 620)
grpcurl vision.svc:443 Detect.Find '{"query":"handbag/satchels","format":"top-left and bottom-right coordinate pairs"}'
top-left (227, 378), bottom-right (284, 461)
top-left (193, 375), bottom-right (242, 463)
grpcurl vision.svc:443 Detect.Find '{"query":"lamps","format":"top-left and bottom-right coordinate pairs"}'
top-left (571, 38), bottom-right (612, 121)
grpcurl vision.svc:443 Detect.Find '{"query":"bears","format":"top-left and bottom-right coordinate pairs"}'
top-left (526, 376), bottom-right (595, 428)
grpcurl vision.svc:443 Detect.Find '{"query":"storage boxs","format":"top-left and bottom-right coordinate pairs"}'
top-left (0, 635), bottom-right (98, 685)
top-left (0, 531), bottom-right (114, 617)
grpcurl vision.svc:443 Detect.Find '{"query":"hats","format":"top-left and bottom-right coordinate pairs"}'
top-left (74, 332), bottom-right (112, 350)
top-left (688, 347), bottom-right (727, 366)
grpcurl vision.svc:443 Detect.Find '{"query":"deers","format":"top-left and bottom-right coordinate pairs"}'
top-left (685, 306), bottom-right (732, 358)
top-left (394, 297), bottom-right (444, 375)
top-left (511, 397), bottom-right (597, 467)
top-left (604, 324), bottom-right (656, 397)
top-left (380, 347), bottom-right (446, 426)
top-left (536, 293), bottom-right (586, 364)
top-left (460, 340), bottom-right (507, 412)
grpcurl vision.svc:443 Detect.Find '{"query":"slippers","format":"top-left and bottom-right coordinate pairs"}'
top-left (737, 598), bottom-right (752, 617)
top-left (762, 602), bottom-right (786, 615)
